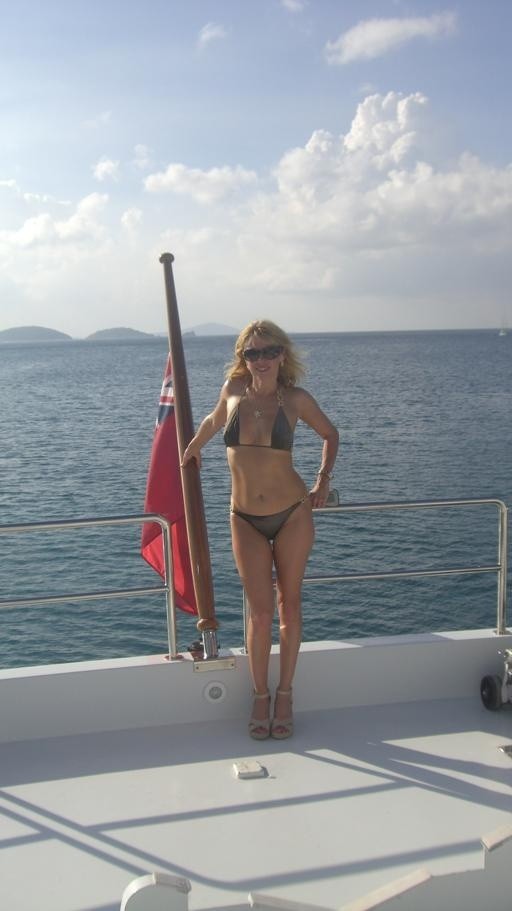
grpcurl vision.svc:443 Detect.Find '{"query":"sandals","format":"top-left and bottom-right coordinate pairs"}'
top-left (270, 685), bottom-right (295, 740)
top-left (248, 687), bottom-right (271, 740)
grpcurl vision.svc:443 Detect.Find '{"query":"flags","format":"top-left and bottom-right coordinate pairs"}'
top-left (139, 350), bottom-right (199, 616)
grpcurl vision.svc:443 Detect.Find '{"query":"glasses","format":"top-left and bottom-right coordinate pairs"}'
top-left (243, 345), bottom-right (284, 362)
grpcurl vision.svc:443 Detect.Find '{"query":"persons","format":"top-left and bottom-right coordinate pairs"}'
top-left (179, 318), bottom-right (341, 742)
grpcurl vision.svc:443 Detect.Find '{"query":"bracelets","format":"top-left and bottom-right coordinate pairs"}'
top-left (318, 469), bottom-right (335, 480)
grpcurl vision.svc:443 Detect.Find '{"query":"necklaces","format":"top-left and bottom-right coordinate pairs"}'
top-left (245, 387), bottom-right (277, 418)
